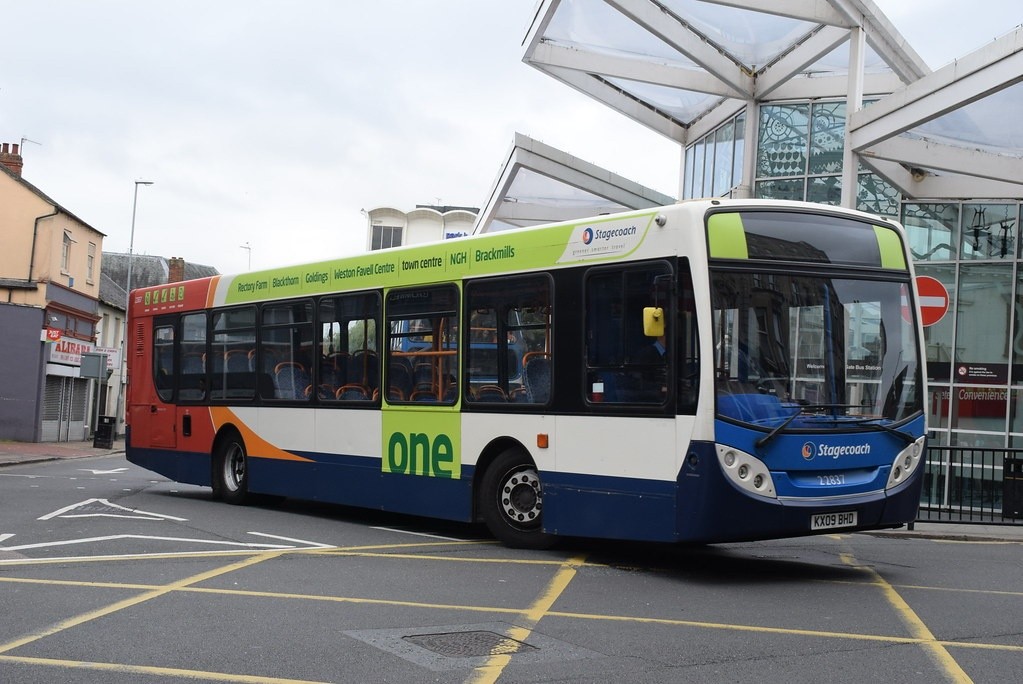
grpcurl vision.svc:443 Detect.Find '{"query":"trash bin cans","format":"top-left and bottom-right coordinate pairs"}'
top-left (93, 416), bottom-right (116, 449)
top-left (1002, 458), bottom-right (1023, 519)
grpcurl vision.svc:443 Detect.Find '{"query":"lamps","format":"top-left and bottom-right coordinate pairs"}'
top-left (95, 329), bottom-right (101, 335)
top-left (999, 204), bottom-right (1015, 259)
top-left (967, 204), bottom-right (991, 252)
top-left (48, 316), bottom-right (59, 324)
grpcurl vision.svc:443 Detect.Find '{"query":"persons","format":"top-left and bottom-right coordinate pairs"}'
top-left (642, 336), bottom-right (669, 360)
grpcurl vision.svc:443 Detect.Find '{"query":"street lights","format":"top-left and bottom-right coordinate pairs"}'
top-left (240, 246), bottom-right (251, 272)
top-left (114, 178), bottom-right (160, 440)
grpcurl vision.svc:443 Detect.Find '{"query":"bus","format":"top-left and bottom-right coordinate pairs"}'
top-left (125, 198), bottom-right (930, 549)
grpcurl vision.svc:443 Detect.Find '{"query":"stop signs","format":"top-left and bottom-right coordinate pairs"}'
top-left (902, 275), bottom-right (949, 326)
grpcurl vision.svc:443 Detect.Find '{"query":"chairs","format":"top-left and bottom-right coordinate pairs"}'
top-left (373, 385), bottom-right (406, 401)
top-left (509, 388), bottom-right (527, 403)
top-left (352, 349), bottom-right (377, 371)
top-left (327, 352), bottom-right (352, 375)
top-left (336, 386), bottom-right (369, 400)
top-left (414, 382), bottom-right (439, 392)
top-left (444, 382), bottom-right (477, 402)
top-left (322, 384), bottom-right (337, 399)
top-left (414, 363), bottom-right (439, 380)
top-left (201, 350), bottom-right (223, 373)
top-left (478, 385), bottom-right (506, 402)
top-left (274, 361), bottom-right (310, 400)
top-left (181, 351), bottom-right (204, 373)
top-left (409, 391), bottom-right (438, 401)
top-left (391, 363), bottom-right (414, 385)
top-left (522, 352), bottom-right (551, 403)
top-left (247, 348), bottom-right (274, 372)
top-left (311, 362), bottom-right (341, 384)
top-left (341, 383), bottom-right (372, 400)
top-left (224, 349), bottom-right (249, 372)
top-left (304, 385), bottom-right (327, 398)
top-left (373, 391), bottom-right (401, 400)
top-left (596, 285), bottom-right (642, 403)
